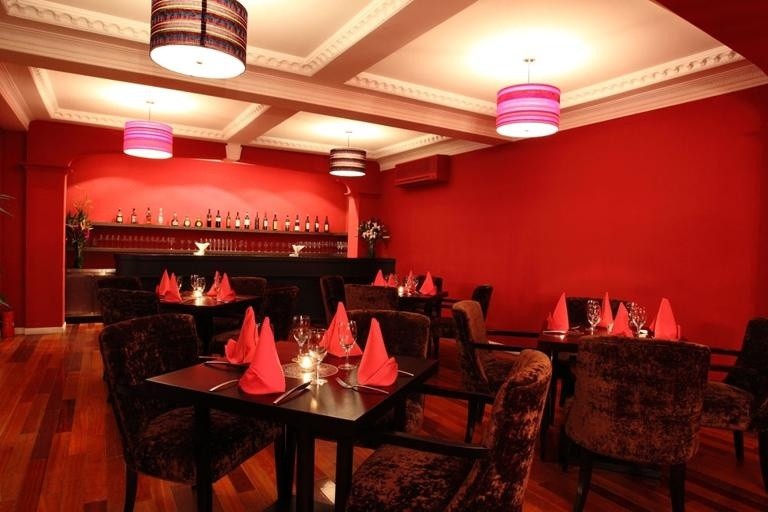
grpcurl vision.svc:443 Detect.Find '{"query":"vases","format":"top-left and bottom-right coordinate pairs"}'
top-left (368, 238), bottom-right (376, 257)
top-left (75, 254), bottom-right (84, 268)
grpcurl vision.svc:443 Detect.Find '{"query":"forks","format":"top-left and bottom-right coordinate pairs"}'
top-left (335, 376), bottom-right (389, 395)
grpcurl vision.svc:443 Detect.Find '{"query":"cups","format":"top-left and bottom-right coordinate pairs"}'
top-left (297, 353), bottom-right (316, 372)
top-left (175, 276), bottom-right (182, 291)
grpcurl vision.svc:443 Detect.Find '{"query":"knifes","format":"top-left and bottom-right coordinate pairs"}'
top-left (543, 330), bottom-right (567, 334)
top-left (203, 360), bottom-right (247, 368)
top-left (273, 380), bottom-right (312, 405)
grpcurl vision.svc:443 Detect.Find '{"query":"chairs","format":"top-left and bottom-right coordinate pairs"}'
top-left (345, 308), bottom-right (431, 436)
top-left (451, 299), bottom-right (557, 461)
top-left (88, 271), bottom-right (301, 339)
top-left (701, 318), bottom-right (768, 491)
top-left (342, 345), bottom-right (554, 511)
top-left (560, 335), bottom-right (709, 510)
top-left (566, 296), bottom-right (632, 333)
top-left (98, 313), bottom-right (293, 510)
top-left (318, 272), bottom-right (493, 340)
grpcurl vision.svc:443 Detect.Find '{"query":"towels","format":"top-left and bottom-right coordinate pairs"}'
top-left (346, 317), bottom-right (400, 386)
top-left (240, 314), bottom-right (287, 397)
top-left (225, 303), bottom-right (262, 363)
top-left (597, 292), bottom-right (684, 341)
top-left (318, 297), bottom-right (364, 356)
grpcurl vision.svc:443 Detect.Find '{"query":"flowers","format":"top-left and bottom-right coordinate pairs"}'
top-left (66, 210), bottom-right (94, 256)
top-left (357, 216), bottom-right (391, 242)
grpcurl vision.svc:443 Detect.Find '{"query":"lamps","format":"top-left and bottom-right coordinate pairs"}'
top-left (122, 98), bottom-right (175, 161)
top-left (149, 0), bottom-right (248, 81)
top-left (494, 56), bottom-right (561, 138)
top-left (329, 129), bottom-right (367, 178)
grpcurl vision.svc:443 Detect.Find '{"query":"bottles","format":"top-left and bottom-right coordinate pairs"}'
top-left (115, 206), bottom-right (329, 232)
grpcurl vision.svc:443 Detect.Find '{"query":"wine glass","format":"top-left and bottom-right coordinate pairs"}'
top-left (627, 302), bottom-right (648, 337)
top-left (307, 327), bottom-right (328, 385)
top-left (91, 233), bottom-right (347, 255)
top-left (190, 275), bottom-right (207, 297)
top-left (292, 314), bottom-right (310, 362)
top-left (338, 319), bottom-right (358, 369)
top-left (384, 273), bottom-right (419, 295)
top-left (586, 300), bottom-right (602, 337)
